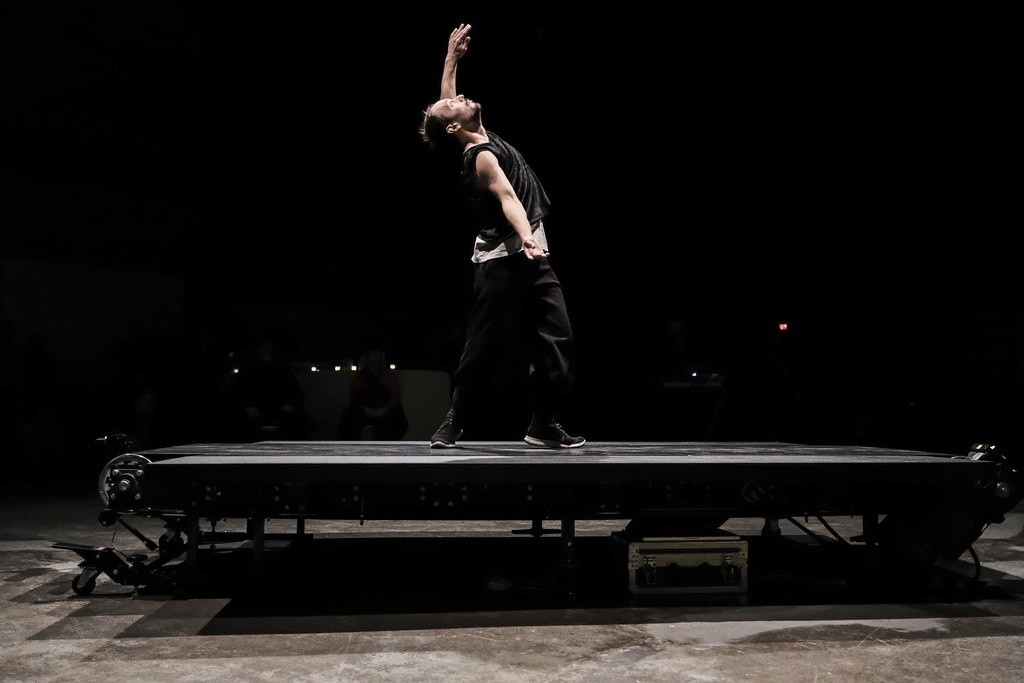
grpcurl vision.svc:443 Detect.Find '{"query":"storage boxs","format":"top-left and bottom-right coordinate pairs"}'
top-left (611, 527), bottom-right (749, 594)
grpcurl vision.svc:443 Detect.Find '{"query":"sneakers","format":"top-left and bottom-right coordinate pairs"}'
top-left (429, 417), bottom-right (464, 449)
top-left (522, 410), bottom-right (586, 449)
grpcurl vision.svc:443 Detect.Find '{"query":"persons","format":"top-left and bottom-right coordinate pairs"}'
top-left (337, 342), bottom-right (409, 441)
top-left (235, 337), bottom-right (311, 440)
top-left (418, 24), bottom-right (588, 448)
